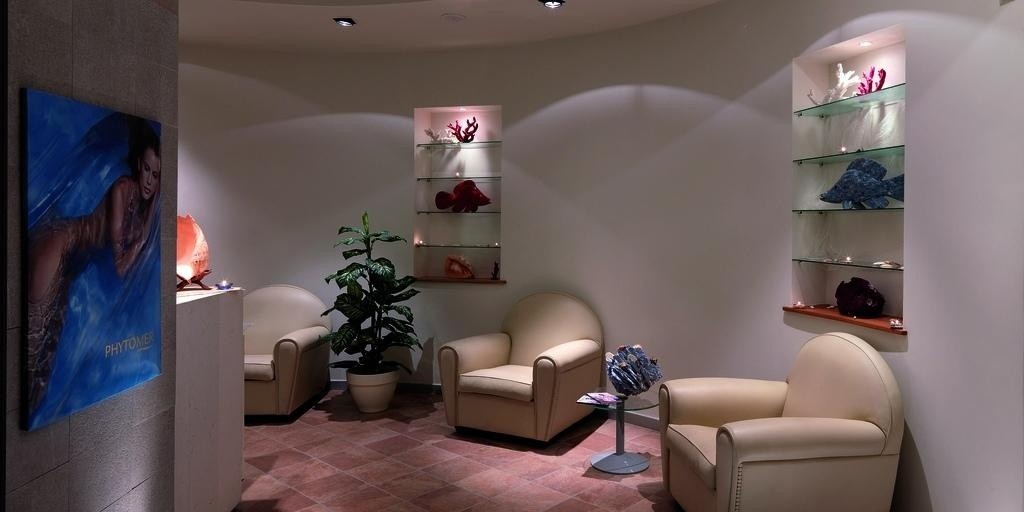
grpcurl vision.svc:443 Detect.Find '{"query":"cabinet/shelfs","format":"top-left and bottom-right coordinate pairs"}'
top-left (780, 20), bottom-right (908, 335)
top-left (410, 104), bottom-right (506, 285)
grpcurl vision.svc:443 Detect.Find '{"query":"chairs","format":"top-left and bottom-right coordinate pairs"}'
top-left (656, 334), bottom-right (905, 511)
top-left (436, 289), bottom-right (605, 451)
top-left (242, 284), bottom-right (334, 423)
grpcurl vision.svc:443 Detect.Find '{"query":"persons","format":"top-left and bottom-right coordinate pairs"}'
top-left (27, 138), bottom-right (161, 419)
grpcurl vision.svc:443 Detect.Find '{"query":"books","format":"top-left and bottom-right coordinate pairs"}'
top-left (576, 392), bottom-right (622, 406)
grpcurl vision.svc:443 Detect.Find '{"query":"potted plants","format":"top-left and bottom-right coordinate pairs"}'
top-left (319, 209), bottom-right (425, 414)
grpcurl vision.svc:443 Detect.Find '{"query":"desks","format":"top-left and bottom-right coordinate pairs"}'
top-left (576, 387), bottom-right (660, 476)
